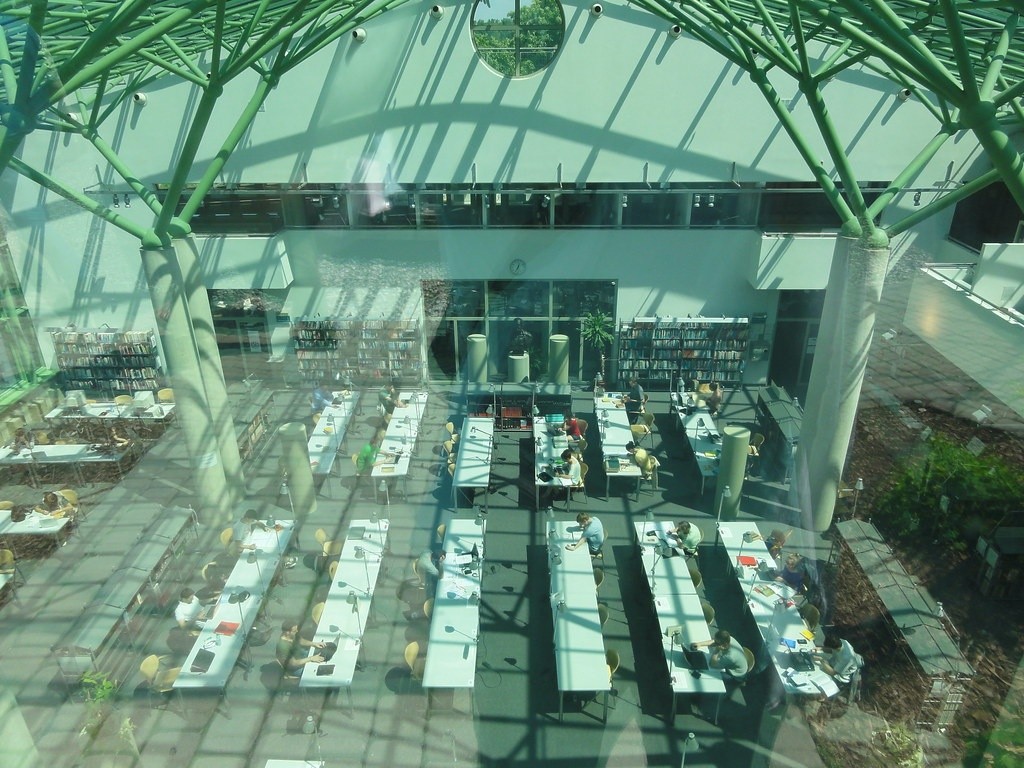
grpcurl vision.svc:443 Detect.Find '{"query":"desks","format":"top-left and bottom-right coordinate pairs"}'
top-left (421, 519), bottom-right (486, 720)
top-left (714, 522), bottom-right (841, 725)
top-left (371, 392), bottom-right (430, 505)
top-left (0, 443), bottom-right (138, 490)
top-left (0, 508), bottom-right (84, 559)
top-left (546, 521), bottom-right (612, 726)
top-left (307, 391), bottom-right (363, 500)
top-left (44, 402), bottom-right (179, 444)
top-left (667, 392), bottom-right (723, 495)
top-left (532, 416), bottom-right (574, 513)
top-left (452, 416), bottom-right (495, 514)
top-left (172, 520), bottom-right (302, 721)
top-left (633, 521), bottom-right (727, 727)
top-left (299, 518), bottom-right (390, 721)
top-left (591, 392), bottom-right (642, 502)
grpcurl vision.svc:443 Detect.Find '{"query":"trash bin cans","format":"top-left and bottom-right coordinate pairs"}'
top-left (266, 353), bottom-right (285, 383)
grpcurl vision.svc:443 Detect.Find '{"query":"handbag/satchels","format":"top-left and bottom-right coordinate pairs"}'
top-left (11, 507), bottom-right (26, 522)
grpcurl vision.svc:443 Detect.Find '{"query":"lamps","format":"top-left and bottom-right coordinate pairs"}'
top-left (484, 195), bottom-right (491, 208)
top-left (708, 195), bottom-right (715, 207)
top-left (124, 193), bottom-right (130, 208)
top-left (333, 194), bottom-right (339, 207)
top-left (914, 192), bottom-right (922, 207)
top-left (694, 194), bottom-right (701, 207)
top-left (622, 195), bottom-right (627, 207)
top-left (408, 195), bottom-right (415, 207)
top-left (542, 194), bottom-right (551, 208)
top-left (114, 194), bottom-right (120, 207)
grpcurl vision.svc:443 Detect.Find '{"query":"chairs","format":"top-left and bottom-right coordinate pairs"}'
top-left (0, 314), bottom-right (862, 768)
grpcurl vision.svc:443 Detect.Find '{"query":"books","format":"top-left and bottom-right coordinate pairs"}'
top-left (618, 323), bottom-right (747, 380)
top-left (52, 331), bottom-right (161, 390)
top-left (294, 320), bottom-right (418, 379)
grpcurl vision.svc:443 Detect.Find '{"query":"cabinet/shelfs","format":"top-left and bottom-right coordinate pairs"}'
top-left (293, 315), bottom-right (427, 390)
top-left (615, 315), bottom-right (749, 392)
top-left (50, 327), bottom-right (167, 402)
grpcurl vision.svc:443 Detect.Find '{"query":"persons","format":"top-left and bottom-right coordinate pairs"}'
top-left (312, 378), bottom-right (337, 423)
top-left (417, 550), bottom-right (446, 585)
top-left (558, 413), bottom-right (580, 441)
top-left (14, 428), bottom-right (39, 449)
top-left (690, 629), bottom-right (748, 684)
top-left (822, 634), bottom-right (858, 689)
top-left (666, 520), bottom-right (701, 555)
top-left (378, 382), bottom-right (409, 414)
top-left (276, 620), bottom-right (326, 677)
top-left (233, 510), bottom-right (265, 554)
top-left (35, 492), bottom-right (73, 548)
top-left (705, 383), bottom-right (722, 413)
top-left (622, 377), bottom-right (645, 422)
top-left (357, 439), bottom-right (395, 474)
top-left (108, 423), bottom-right (131, 443)
top-left (546, 449), bottom-right (581, 496)
top-left (175, 589), bottom-right (206, 631)
top-left (626, 441), bottom-right (651, 478)
top-left (566, 513), bottom-right (604, 555)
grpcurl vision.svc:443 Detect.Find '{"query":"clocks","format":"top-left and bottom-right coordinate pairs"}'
top-left (509, 258), bottom-right (528, 277)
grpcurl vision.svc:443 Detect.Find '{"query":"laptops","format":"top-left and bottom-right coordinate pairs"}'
top-left (548, 429), bottom-right (565, 436)
top-left (681, 643), bottom-right (709, 670)
top-left (757, 560), bottom-right (779, 581)
top-left (463, 543), bottom-right (479, 566)
top-left (312, 634), bottom-right (340, 662)
top-left (40, 518), bottom-right (58, 528)
top-left (787, 642), bottom-right (815, 673)
top-left (385, 455), bottom-right (401, 463)
top-left (333, 395), bottom-right (344, 405)
top-left (704, 427), bottom-right (722, 445)
top-left (191, 648), bottom-right (215, 673)
top-left (549, 464), bottom-right (563, 476)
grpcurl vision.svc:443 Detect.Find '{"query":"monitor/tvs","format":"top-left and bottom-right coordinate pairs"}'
top-left (132, 391), bottom-right (155, 408)
top-left (64, 389), bottom-right (87, 408)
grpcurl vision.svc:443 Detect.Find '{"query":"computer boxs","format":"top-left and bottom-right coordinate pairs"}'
top-left (132, 405), bottom-right (160, 417)
top-left (63, 404), bottom-right (91, 415)
